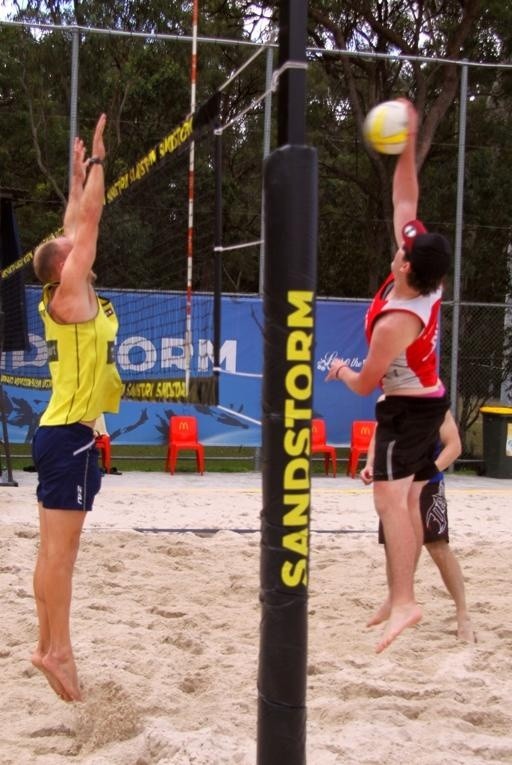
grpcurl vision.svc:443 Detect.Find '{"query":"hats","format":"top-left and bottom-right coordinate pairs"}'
top-left (403, 220), bottom-right (451, 281)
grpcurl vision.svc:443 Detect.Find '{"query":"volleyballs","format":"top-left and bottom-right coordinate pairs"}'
top-left (363, 101), bottom-right (413, 157)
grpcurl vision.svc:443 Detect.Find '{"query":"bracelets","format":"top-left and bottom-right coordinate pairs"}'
top-left (86, 156), bottom-right (106, 166)
top-left (335, 362), bottom-right (348, 377)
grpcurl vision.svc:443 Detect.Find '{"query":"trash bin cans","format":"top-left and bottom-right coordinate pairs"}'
top-left (476, 406), bottom-right (512, 479)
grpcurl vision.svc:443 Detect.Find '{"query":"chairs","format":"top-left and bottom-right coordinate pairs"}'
top-left (165, 416), bottom-right (204, 475)
top-left (347, 421), bottom-right (377, 479)
top-left (311, 418), bottom-right (337, 478)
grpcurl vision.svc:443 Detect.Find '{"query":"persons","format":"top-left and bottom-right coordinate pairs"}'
top-left (323, 95), bottom-right (452, 657)
top-left (31, 112), bottom-right (125, 705)
top-left (360, 394), bottom-right (477, 647)
